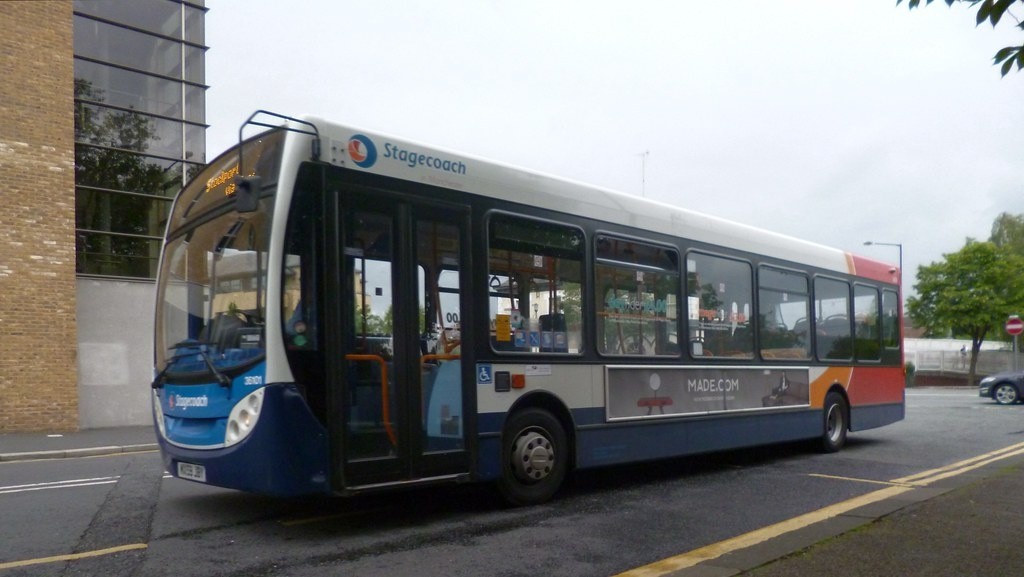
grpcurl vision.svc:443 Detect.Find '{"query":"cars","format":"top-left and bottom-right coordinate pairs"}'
top-left (978, 369), bottom-right (1024, 405)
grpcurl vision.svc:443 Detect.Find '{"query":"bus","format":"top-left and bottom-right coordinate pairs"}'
top-left (148, 110), bottom-right (905, 508)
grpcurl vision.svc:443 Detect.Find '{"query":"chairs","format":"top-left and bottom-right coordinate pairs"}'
top-left (354, 310), bottom-right (900, 420)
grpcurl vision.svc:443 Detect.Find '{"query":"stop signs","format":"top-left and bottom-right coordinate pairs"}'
top-left (1006, 316), bottom-right (1024, 336)
